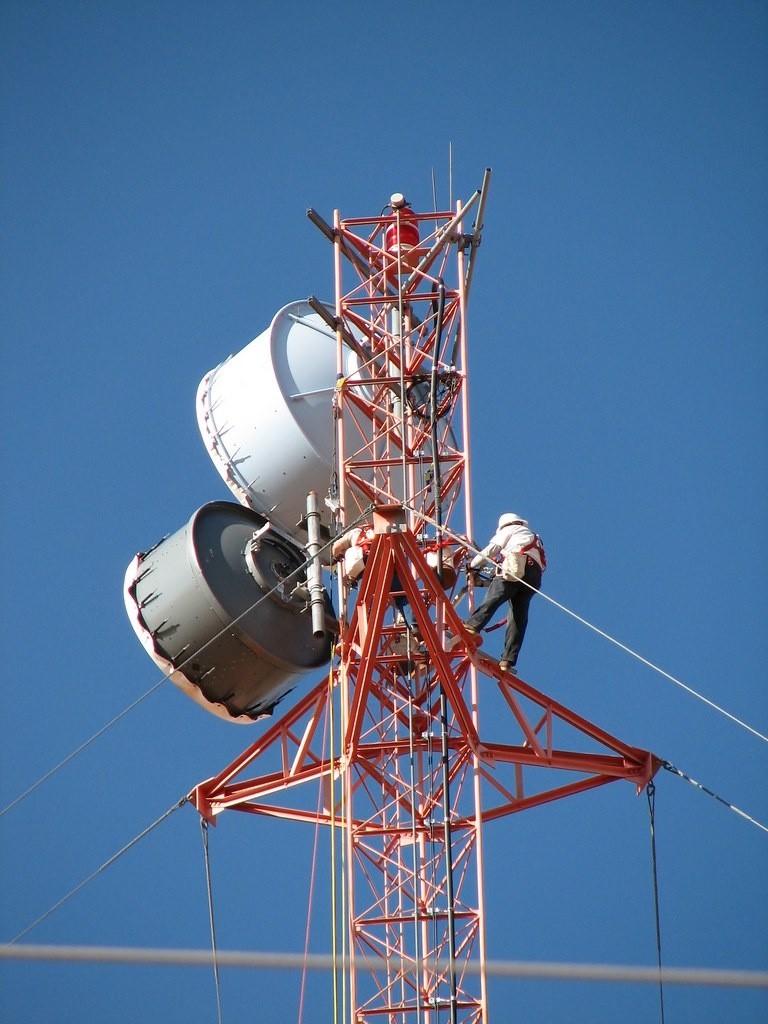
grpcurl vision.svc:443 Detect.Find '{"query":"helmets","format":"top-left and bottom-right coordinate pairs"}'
top-left (496, 513), bottom-right (528, 533)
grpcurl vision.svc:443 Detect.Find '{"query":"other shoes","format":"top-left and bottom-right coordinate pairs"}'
top-left (461, 621), bottom-right (478, 635)
top-left (499, 660), bottom-right (512, 672)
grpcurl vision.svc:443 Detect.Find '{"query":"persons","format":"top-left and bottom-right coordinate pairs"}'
top-left (448, 513), bottom-right (546, 671)
top-left (333, 525), bottom-right (408, 579)
